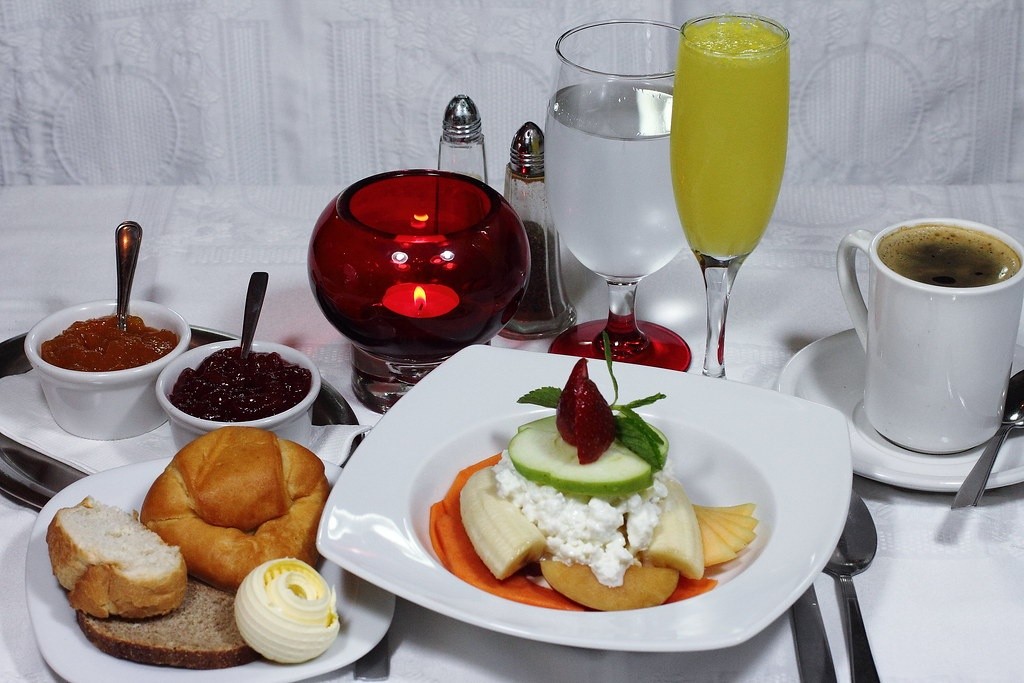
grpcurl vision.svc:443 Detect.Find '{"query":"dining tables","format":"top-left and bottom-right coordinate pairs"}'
top-left (1, 182), bottom-right (1024, 683)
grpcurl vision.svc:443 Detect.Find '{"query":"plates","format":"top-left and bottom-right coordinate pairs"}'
top-left (27, 462), bottom-right (396, 683)
top-left (315, 345), bottom-right (853, 653)
top-left (776, 328), bottom-right (1024, 493)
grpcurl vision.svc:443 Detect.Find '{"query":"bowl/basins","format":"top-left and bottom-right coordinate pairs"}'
top-left (25, 299), bottom-right (191, 439)
top-left (156, 339), bottom-right (322, 454)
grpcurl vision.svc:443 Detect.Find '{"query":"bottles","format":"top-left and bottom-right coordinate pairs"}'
top-left (434, 94), bottom-right (490, 246)
top-left (493, 120), bottom-right (578, 341)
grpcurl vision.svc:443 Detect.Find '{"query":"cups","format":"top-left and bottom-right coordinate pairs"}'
top-left (838, 217), bottom-right (1024, 454)
top-left (672, 13), bottom-right (790, 377)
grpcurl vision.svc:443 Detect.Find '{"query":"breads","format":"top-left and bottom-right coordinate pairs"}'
top-left (76, 578), bottom-right (261, 669)
top-left (138, 425), bottom-right (330, 593)
top-left (47, 496), bottom-right (188, 619)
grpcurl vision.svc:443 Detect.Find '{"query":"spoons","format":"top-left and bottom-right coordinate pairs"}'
top-left (822, 492), bottom-right (879, 683)
top-left (950, 370), bottom-right (1024, 507)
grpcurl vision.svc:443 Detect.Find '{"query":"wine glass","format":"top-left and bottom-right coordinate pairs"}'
top-left (545, 18), bottom-right (692, 370)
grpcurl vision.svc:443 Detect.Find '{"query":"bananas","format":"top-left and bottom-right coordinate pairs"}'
top-left (646, 483), bottom-right (705, 580)
top-left (458, 466), bottom-right (549, 580)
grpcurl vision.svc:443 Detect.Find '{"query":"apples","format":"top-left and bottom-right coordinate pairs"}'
top-left (508, 413), bottom-right (670, 494)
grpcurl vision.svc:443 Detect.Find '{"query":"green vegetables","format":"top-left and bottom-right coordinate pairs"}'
top-left (517, 329), bottom-right (666, 470)
